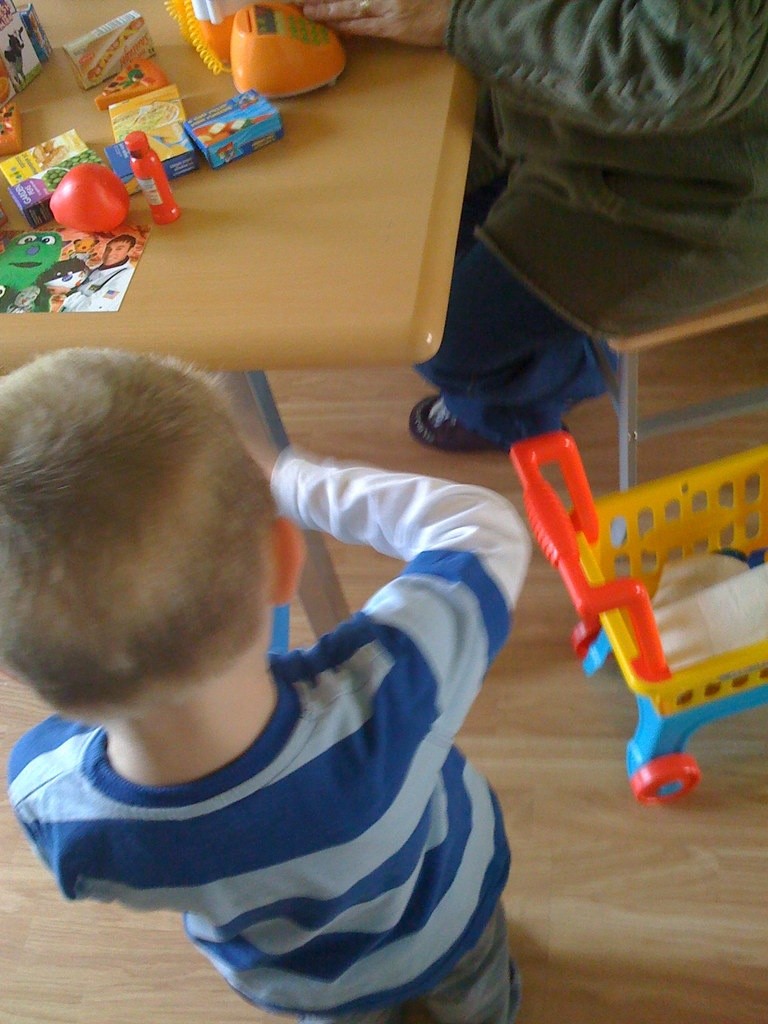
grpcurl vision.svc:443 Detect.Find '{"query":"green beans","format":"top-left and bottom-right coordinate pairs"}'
top-left (43, 148), bottom-right (106, 192)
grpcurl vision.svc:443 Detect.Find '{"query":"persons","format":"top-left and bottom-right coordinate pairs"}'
top-left (0, 349), bottom-right (530, 1024)
top-left (59, 235), bottom-right (134, 312)
top-left (301, 0), bottom-right (768, 451)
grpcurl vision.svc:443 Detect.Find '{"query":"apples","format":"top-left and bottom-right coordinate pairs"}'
top-left (49, 162), bottom-right (131, 232)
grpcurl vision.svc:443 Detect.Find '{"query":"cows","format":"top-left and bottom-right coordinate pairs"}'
top-left (4, 26), bottom-right (26, 86)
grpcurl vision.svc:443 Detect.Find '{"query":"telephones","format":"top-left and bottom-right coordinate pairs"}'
top-left (172, 0), bottom-right (348, 99)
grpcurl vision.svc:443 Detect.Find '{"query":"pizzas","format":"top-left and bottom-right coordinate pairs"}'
top-left (0, 102), bottom-right (24, 156)
top-left (95, 58), bottom-right (169, 111)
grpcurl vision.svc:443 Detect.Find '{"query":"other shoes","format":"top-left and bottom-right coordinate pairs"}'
top-left (408, 395), bottom-right (570, 454)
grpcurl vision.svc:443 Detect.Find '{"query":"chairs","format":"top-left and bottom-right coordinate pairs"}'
top-left (596, 290), bottom-right (766, 495)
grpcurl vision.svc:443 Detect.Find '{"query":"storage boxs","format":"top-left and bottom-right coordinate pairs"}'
top-left (0, 102), bottom-right (106, 230)
top-left (104, 121), bottom-right (198, 195)
top-left (182, 89), bottom-right (283, 170)
top-left (62, 10), bottom-right (158, 90)
top-left (0, 0), bottom-right (52, 102)
top-left (108, 83), bottom-right (187, 144)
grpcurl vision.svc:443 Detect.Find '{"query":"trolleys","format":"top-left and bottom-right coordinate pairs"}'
top-left (511, 432), bottom-right (767, 808)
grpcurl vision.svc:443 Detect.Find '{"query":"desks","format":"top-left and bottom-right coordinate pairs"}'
top-left (0, 0), bottom-right (483, 639)
